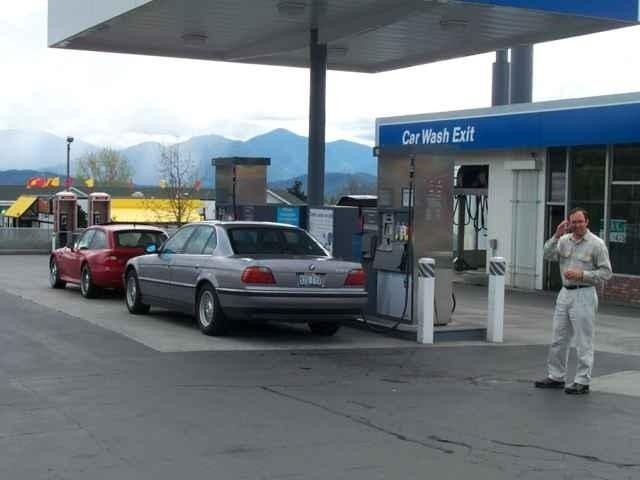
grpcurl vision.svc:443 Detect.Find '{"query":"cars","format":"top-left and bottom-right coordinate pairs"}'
top-left (121, 219), bottom-right (369, 337)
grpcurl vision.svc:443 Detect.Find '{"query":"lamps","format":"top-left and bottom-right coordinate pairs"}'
top-left (441, 19), bottom-right (468, 35)
top-left (276, 2), bottom-right (305, 18)
top-left (181, 33), bottom-right (209, 49)
top-left (326, 47), bottom-right (347, 57)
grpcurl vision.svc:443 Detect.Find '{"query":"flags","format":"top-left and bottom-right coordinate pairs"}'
top-left (194, 179), bottom-right (201, 191)
top-left (159, 179), bottom-right (166, 190)
top-left (25, 175), bottom-right (74, 190)
top-left (85, 177), bottom-right (94, 188)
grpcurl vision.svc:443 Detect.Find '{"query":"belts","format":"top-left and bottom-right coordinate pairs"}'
top-left (563, 284), bottom-right (595, 290)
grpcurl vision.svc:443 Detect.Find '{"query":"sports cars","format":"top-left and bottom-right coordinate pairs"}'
top-left (49, 224), bottom-right (170, 299)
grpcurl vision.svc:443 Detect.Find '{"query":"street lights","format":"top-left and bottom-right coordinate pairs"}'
top-left (66, 135), bottom-right (74, 176)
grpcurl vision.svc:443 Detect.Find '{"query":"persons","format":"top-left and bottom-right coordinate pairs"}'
top-left (533, 207), bottom-right (612, 395)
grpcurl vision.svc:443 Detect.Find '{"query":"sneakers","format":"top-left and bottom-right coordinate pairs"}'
top-left (564, 382), bottom-right (590, 395)
top-left (534, 376), bottom-right (566, 389)
